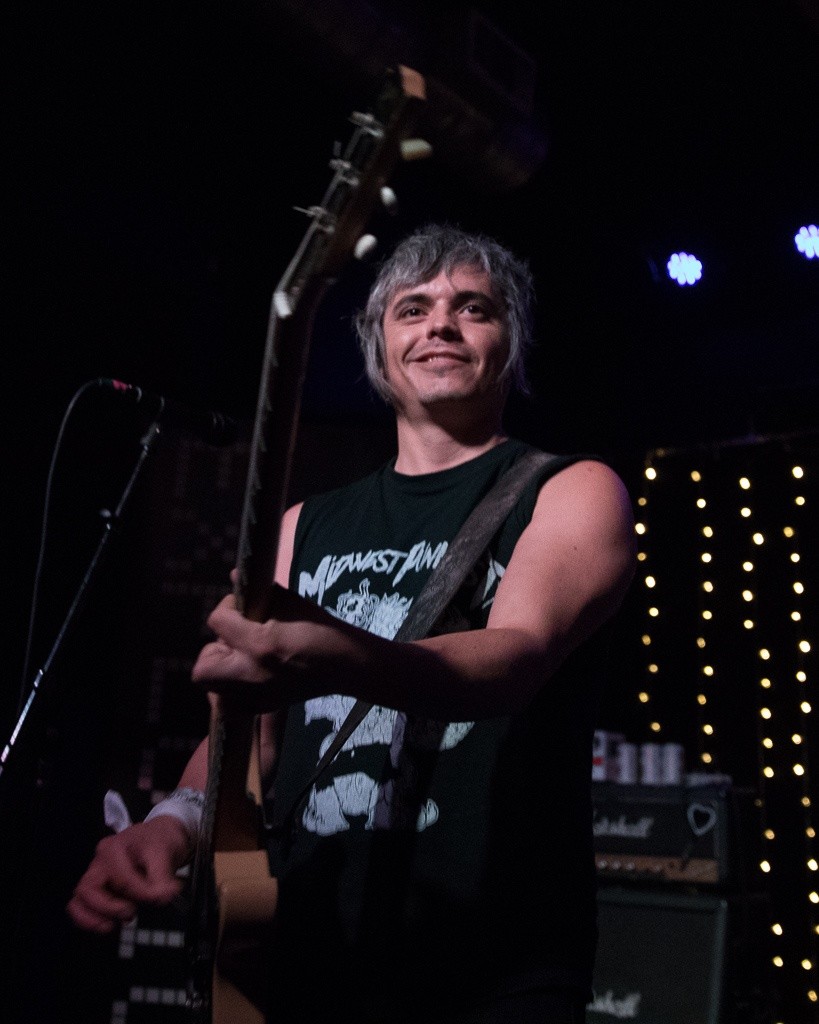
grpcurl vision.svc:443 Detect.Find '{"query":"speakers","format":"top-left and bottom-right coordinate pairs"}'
top-left (582, 891), bottom-right (729, 1024)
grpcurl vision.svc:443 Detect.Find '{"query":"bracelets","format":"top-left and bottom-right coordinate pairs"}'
top-left (142, 789), bottom-right (208, 844)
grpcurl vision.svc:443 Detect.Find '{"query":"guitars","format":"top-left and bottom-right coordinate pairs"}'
top-left (187, 57), bottom-right (428, 1023)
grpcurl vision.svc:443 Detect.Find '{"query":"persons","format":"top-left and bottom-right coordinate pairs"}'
top-left (69, 223), bottom-right (638, 1024)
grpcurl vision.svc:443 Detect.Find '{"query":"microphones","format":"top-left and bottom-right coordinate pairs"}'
top-left (95, 381), bottom-right (237, 434)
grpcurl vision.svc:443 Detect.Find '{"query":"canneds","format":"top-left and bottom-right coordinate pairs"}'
top-left (615, 741), bottom-right (686, 785)
